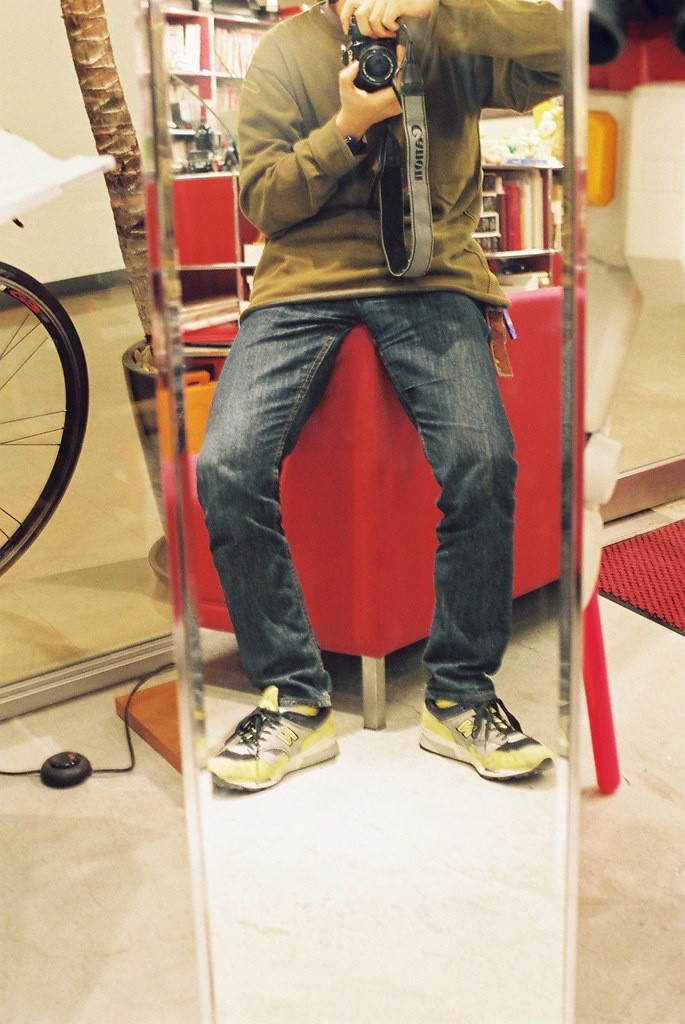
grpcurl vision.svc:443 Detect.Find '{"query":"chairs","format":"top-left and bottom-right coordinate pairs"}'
top-left (162, 285), bottom-right (585, 731)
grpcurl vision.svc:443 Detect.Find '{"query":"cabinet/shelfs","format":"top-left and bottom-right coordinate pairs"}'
top-left (476, 160), bottom-right (564, 261)
top-left (162, 9), bottom-right (275, 171)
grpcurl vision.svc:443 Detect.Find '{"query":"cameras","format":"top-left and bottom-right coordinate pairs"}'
top-left (340, 18), bottom-right (397, 93)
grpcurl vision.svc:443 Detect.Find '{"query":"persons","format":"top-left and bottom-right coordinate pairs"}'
top-left (196, 0), bottom-right (571, 792)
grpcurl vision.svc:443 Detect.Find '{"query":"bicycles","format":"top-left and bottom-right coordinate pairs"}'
top-left (2, 260), bottom-right (89, 581)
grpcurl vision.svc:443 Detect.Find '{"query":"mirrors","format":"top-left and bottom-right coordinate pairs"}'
top-left (147, 0), bottom-right (590, 1023)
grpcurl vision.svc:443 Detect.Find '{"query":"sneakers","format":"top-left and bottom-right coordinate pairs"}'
top-left (209, 685), bottom-right (339, 791)
top-left (419, 689), bottom-right (555, 781)
